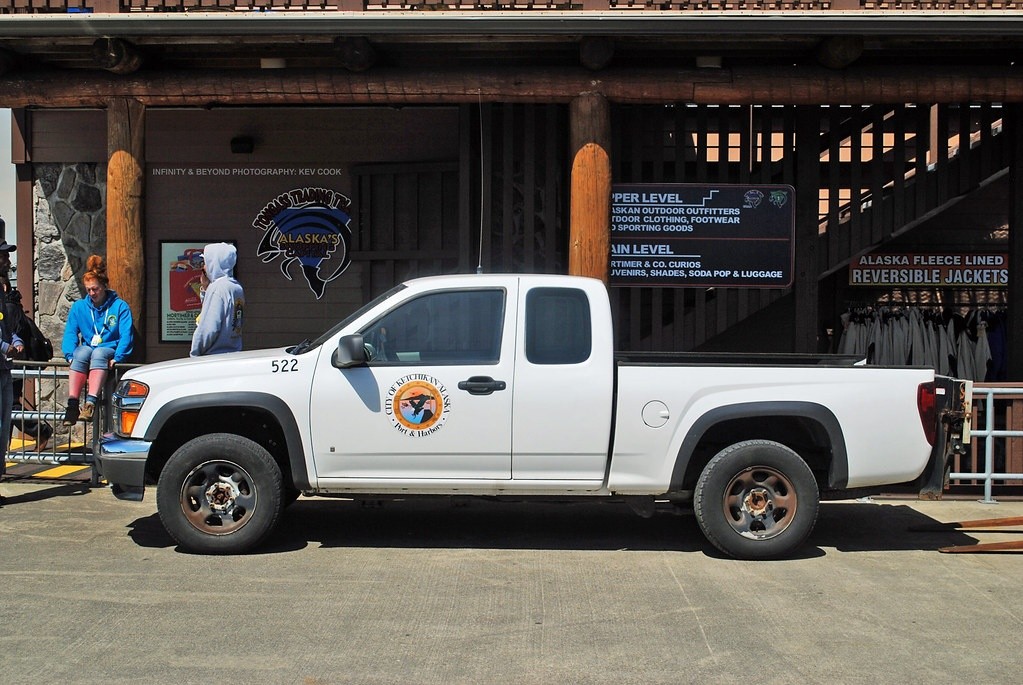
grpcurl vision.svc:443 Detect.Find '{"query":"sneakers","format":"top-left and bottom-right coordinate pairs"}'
top-left (63, 405), bottom-right (80, 425)
top-left (33, 424), bottom-right (52, 452)
top-left (78, 403), bottom-right (95, 421)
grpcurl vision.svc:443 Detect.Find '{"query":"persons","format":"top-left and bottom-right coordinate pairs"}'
top-left (0, 218), bottom-right (53, 510)
top-left (190, 242), bottom-right (246, 357)
top-left (61, 254), bottom-right (133, 427)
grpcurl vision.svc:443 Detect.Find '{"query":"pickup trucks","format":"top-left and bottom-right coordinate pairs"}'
top-left (95, 274), bottom-right (972, 562)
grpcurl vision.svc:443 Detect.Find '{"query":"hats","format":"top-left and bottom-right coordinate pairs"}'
top-left (0, 243), bottom-right (16, 252)
top-left (199, 248), bottom-right (205, 258)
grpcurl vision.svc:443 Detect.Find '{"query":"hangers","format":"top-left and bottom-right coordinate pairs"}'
top-left (844, 301), bottom-right (991, 326)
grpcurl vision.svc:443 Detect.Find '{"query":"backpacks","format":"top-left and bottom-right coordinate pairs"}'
top-left (6, 300), bottom-right (53, 370)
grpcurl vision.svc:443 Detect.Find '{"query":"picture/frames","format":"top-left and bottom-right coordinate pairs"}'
top-left (158, 239), bottom-right (237, 344)
